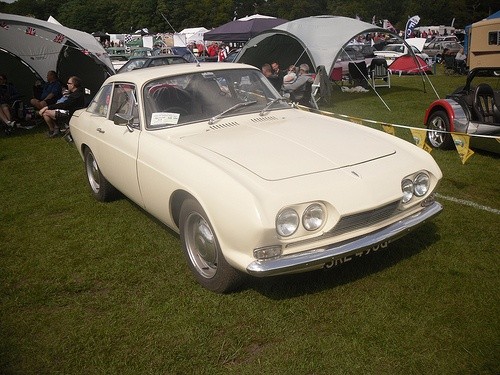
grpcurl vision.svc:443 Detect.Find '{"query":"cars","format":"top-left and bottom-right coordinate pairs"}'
top-left (422, 40), bottom-right (464, 64)
top-left (373, 36), bottom-right (430, 68)
top-left (423, 33), bottom-right (464, 49)
top-left (68, 61), bottom-right (444, 295)
top-left (106, 45), bottom-right (246, 88)
top-left (333, 42), bottom-right (378, 74)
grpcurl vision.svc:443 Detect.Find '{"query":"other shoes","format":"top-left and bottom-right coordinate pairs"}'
top-left (7, 120), bottom-right (17, 128)
top-left (45, 126), bottom-right (61, 138)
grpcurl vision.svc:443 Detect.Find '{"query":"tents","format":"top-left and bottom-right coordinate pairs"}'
top-left (464, 10), bottom-right (500, 74)
top-left (0, 12), bottom-right (129, 108)
top-left (178, 27), bottom-right (223, 46)
top-left (203, 12), bottom-right (289, 56)
top-left (232, 15), bottom-right (442, 113)
top-left (388, 54), bottom-right (433, 76)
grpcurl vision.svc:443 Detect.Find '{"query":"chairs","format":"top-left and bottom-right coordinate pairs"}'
top-left (367, 59), bottom-right (391, 90)
top-left (472, 82), bottom-right (500, 125)
top-left (285, 77), bottom-right (314, 108)
top-left (444, 54), bottom-right (461, 77)
top-left (152, 86), bottom-right (203, 117)
top-left (329, 66), bottom-right (344, 90)
top-left (311, 65), bottom-right (333, 109)
top-left (348, 61), bottom-right (370, 90)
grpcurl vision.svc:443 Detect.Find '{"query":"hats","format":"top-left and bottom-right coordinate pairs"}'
top-left (283, 75), bottom-right (297, 84)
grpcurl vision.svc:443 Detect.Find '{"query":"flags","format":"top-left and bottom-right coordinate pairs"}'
top-left (405, 15), bottom-right (420, 39)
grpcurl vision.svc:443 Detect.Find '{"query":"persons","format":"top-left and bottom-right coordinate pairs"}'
top-left (357, 28), bottom-right (448, 41)
top-left (236, 62), bottom-right (310, 102)
top-left (29, 70), bottom-right (63, 108)
top-left (99, 38), bottom-right (124, 51)
top-left (37, 75), bottom-right (88, 138)
top-left (443, 48), bottom-right (469, 73)
top-left (0, 74), bottom-right (45, 128)
top-left (189, 41), bottom-right (232, 62)
